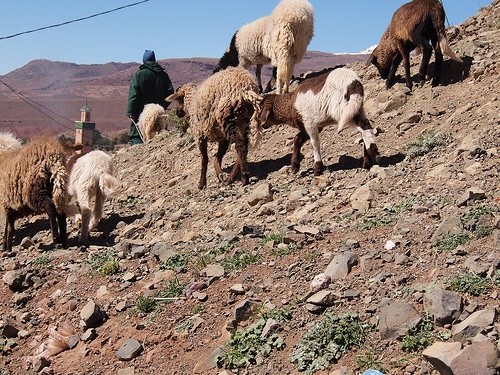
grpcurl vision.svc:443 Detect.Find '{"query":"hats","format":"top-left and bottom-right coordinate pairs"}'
top-left (142, 50), bottom-right (155, 64)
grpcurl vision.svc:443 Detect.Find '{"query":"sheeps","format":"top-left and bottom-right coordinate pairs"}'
top-left (1, 128), bottom-right (123, 254)
top-left (132, 1), bottom-right (467, 192)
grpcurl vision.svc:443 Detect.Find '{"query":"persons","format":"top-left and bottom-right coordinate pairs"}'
top-left (126, 50), bottom-right (174, 146)
